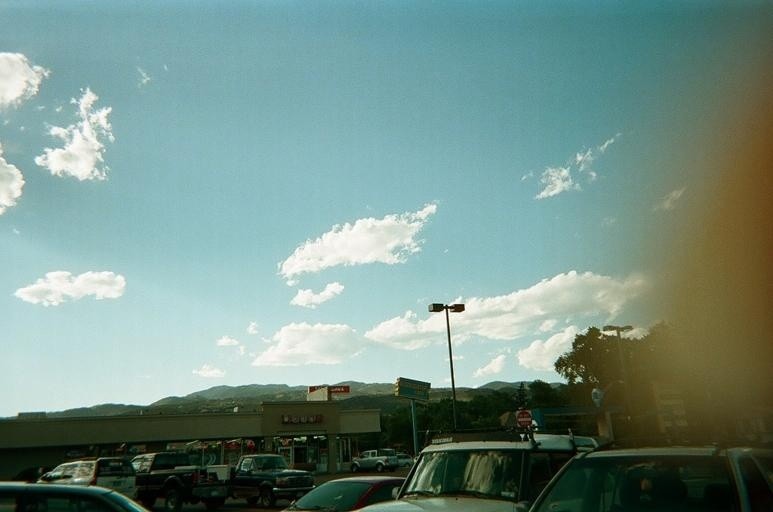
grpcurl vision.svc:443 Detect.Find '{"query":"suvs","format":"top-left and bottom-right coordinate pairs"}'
top-left (529, 439), bottom-right (771, 512)
top-left (36, 456), bottom-right (137, 511)
top-left (353, 430), bottom-right (622, 511)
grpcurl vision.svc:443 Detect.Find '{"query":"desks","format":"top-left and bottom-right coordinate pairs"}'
top-left (602, 323), bottom-right (633, 412)
top-left (428, 301), bottom-right (466, 427)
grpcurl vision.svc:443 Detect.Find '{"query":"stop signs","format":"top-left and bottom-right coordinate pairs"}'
top-left (514, 409), bottom-right (531, 430)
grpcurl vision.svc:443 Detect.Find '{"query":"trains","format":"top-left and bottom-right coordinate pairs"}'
top-left (514, 409), bottom-right (531, 430)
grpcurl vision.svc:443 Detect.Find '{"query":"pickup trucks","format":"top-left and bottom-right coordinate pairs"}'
top-left (351, 449), bottom-right (399, 474)
top-left (121, 451), bottom-right (237, 512)
top-left (226, 454), bottom-right (316, 511)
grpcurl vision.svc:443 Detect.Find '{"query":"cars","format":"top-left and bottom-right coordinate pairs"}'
top-left (396, 454), bottom-right (414, 468)
top-left (1, 481), bottom-right (150, 512)
top-left (282, 475), bottom-right (408, 512)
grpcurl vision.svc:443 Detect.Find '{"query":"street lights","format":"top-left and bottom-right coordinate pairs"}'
top-left (602, 323), bottom-right (633, 412)
top-left (428, 301), bottom-right (466, 427)
